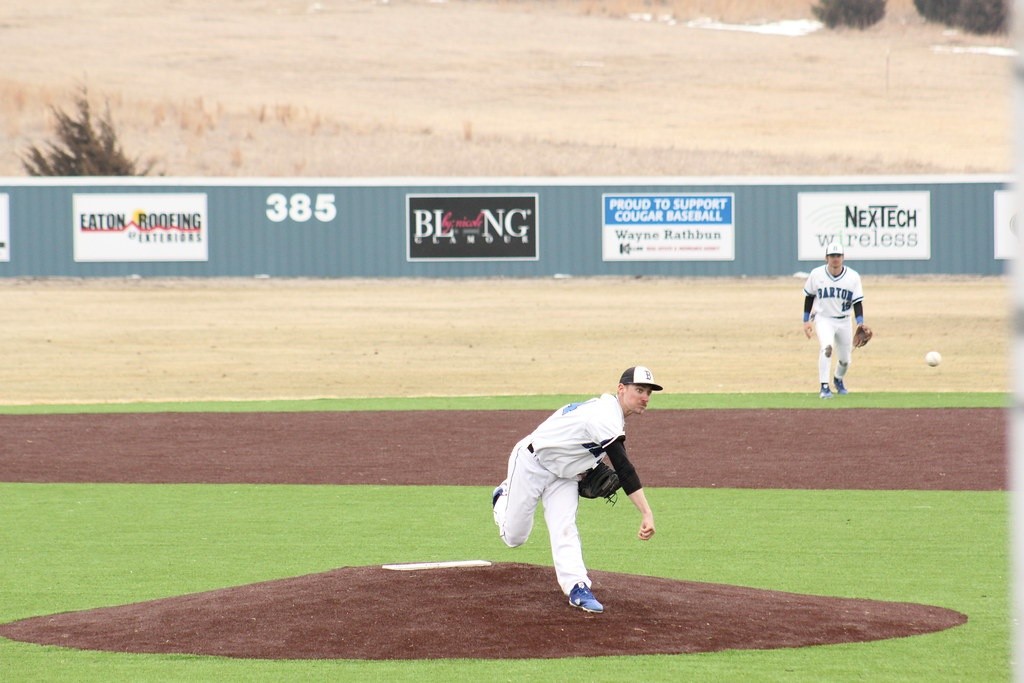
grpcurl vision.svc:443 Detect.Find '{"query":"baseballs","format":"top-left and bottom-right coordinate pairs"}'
top-left (926, 352), bottom-right (942, 366)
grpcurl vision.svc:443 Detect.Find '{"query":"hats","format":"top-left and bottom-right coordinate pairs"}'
top-left (826, 241), bottom-right (844, 256)
top-left (619, 366), bottom-right (663, 391)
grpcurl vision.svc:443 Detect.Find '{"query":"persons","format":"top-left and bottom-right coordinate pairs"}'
top-left (489, 365), bottom-right (663, 613)
top-left (802, 242), bottom-right (865, 398)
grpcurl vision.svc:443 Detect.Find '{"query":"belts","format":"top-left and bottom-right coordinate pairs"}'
top-left (527, 444), bottom-right (536, 456)
top-left (831, 315), bottom-right (846, 319)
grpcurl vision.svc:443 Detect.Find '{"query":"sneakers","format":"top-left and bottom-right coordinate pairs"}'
top-left (820, 382), bottom-right (832, 398)
top-left (493, 478), bottom-right (508, 498)
top-left (834, 375), bottom-right (847, 394)
top-left (569, 582), bottom-right (603, 613)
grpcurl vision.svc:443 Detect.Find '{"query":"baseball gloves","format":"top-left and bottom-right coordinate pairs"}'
top-left (853, 324), bottom-right (872, 347)
top-left (579, 462), bottom-right (622, 499)
